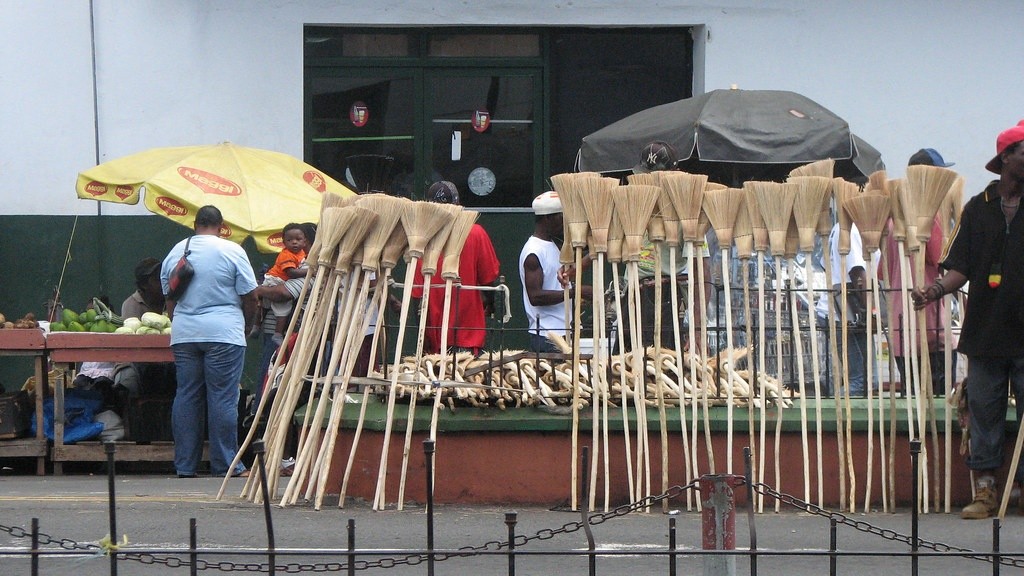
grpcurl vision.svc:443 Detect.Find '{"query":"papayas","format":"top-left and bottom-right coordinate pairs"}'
top-left (49, 308), bottom-right (116, 332)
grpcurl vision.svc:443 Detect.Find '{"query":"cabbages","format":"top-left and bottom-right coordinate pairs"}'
top-left (115, 312), bottom-right (171, 335)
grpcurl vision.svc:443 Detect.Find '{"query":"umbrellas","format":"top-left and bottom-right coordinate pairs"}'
top-left (574, 84), bottom-right (887, 181)
top-left (75, 141), bottom-right (368, 253)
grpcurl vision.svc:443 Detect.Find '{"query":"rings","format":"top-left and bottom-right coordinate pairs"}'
top-left (912, 300), bottom-right (915, 304)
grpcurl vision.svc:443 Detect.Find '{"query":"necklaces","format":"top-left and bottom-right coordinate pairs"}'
top-left (999, 187), bottom-right (1022, 231)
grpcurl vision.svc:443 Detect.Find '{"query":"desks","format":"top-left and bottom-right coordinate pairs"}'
top-left (0, 329), bottom-right (46, 475)
top-left (45, 331), bottom-right (212, 475)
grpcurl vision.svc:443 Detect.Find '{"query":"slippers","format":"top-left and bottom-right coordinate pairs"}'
top-left (178, 475), bottom-right (197, 478)
top-left (231, 469), bottom-right (251, 478)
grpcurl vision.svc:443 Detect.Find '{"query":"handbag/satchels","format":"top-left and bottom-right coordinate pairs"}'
top-left (169, 236), bottom-right (194, 301)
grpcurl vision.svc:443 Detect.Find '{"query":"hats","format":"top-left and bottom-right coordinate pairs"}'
top-left (908, 148), bottom-right (956, 168)
top-left (135, 257), bottom-right (163, 282)
top-left (632, 140), bottom-right (679, 174)
top-left (428, 181), bottom-right (460, 204)
top-left (985, 126), bottom-right (1024, 175)
top-left (532, 191), bottom-right (564, 215)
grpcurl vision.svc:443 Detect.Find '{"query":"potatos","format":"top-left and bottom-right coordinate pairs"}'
top-left (0, 313), bottom-right (40, 329)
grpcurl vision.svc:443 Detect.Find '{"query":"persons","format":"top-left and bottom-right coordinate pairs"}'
top-left (817, 146), bottom-right (954, 399)
top-left (911, 120), bottom-right (1024, 519)
top-left (253, 223), bottom-right (337, 477)
top-left (397, 180), bottom-right (502, 361)
top-left (160, 205), bottom-right (258, 478)
top-left (120, 257), bottom-right (177, 442)
top-left (518, 141), bottom-right (711, 359)
top-left (329, 261), bottom-right (387, 385)
top-left (73, 295), bottom-right (114, 408)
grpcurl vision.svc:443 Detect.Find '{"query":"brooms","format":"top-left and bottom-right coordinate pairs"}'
top-left (217, 191), bottom-right (482, 513)
top-left (547, 157), bottom-right (964, 518)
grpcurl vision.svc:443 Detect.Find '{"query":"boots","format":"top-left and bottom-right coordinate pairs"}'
top-left (961, 479), bottom-right (998, 518)
